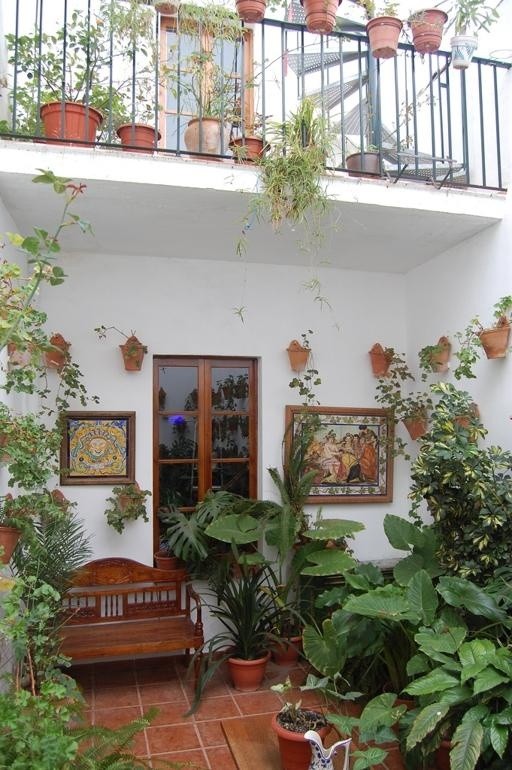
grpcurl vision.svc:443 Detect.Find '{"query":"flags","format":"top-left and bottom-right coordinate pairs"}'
top-left (45, 332), bottom-right (73, 369)
top-left (116, 335), bottom-right (147, 372)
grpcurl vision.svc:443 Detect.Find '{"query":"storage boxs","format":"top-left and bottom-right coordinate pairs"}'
top-left (27, 556), bottom-right (204, 702)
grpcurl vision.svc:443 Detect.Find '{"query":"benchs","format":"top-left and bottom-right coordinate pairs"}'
top-left (27, 556), bottom-right (204, 702)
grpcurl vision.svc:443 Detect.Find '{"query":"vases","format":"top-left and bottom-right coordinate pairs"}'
top-left (45, 332), bottom-right (73, 369)
top-left (116, 335), bottom-right (147, 372)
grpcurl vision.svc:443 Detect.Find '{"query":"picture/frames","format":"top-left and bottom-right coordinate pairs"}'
top-left (59, 409), bottom-right (137, 486)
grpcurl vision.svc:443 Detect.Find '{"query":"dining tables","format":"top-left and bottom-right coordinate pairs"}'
top-left (0, 491), bottom-right (67, 566)
top-left (40, 675), bottom-right (81, 730)
top-left (155, 550), bottom-right (180, 569)
top-left (103, 480), bottom-right (152, 535)
top-left (1, 404), bottom-right (62, 492)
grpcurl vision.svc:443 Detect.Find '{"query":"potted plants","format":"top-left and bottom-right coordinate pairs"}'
top-left (155, 550), bottom-right (180, 569)
top-left (419, 335), bottom-right (450, 384)
top-left (407, 9), bottom-right (450, 54)
top-left (40, 675), bottom-right (81, 730)
top-left (289, 338), bottom-right (323, 405)
top-left (59, 409), bottom-right (137, 486)
top-left (231, 61), bottom-right (437, 260)
top-left (0, 491), bottom-right (67, 566)
top-left (5, 3), bottom-right (238, 159)
top-left (103, 480), bottom-right (152, 535)
top-left (185, 383), bottom-right (510, 770)
top-left (451, 0), bottom-right (500, 69)
top-left (300, 0), bottom-right (343, 34)
top-left (363, 0), bottom-right (403, 60)
top-left (370, 343), bottom-right (413, 408)
top-left (236, 0), bottom-right (283, 24)
top-left (1, 404), bottom-right (62, 492)
top-left (454, 301), bottom-right (512, 380)
